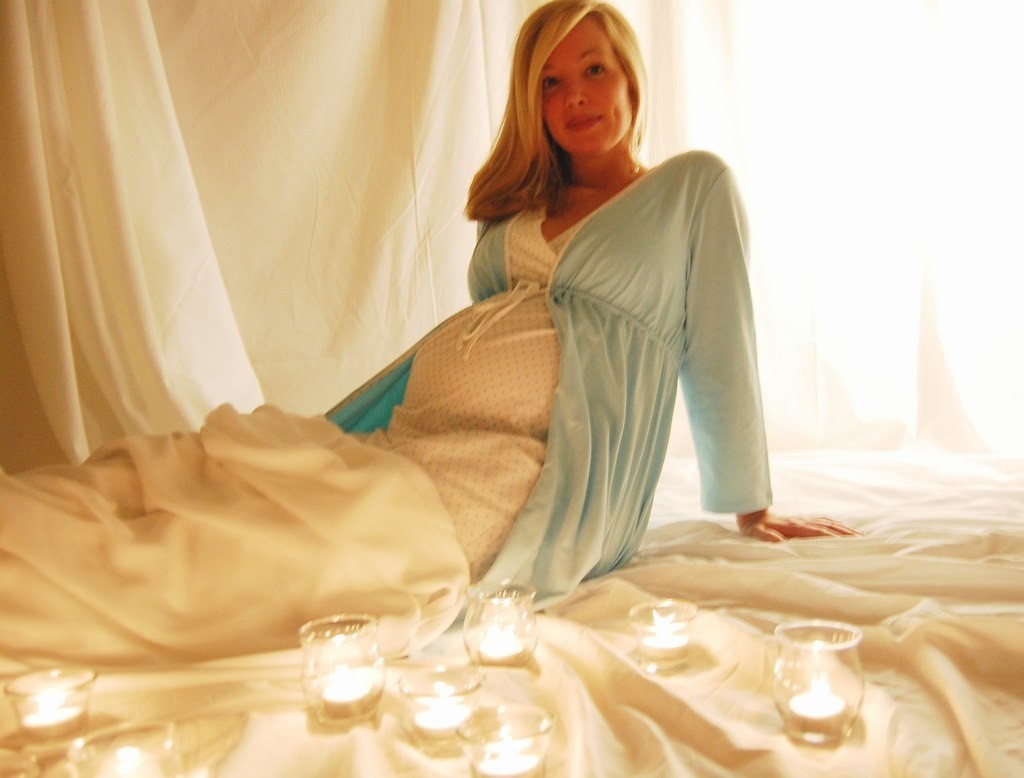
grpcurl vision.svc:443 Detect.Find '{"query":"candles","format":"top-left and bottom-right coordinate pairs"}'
top-left (4, 664), bottom-right (100, 765)
top-left (768, 617), bottom-right (865, 750)
top-left (455, 704), bottom-right (558, 777)
top-left (461, 578), bottom-right (540, 669)
top-left (296, 614), bottom-right (387, 728)
top-left (66, 717), bottom-right (184, 778)
top-left (395, 663), bottom-right (487, 757)
top-left (625, 598), bottom-right (699, 678)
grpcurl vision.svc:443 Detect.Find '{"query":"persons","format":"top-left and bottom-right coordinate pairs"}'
top-left (321, 2), bottom-right (865, 624)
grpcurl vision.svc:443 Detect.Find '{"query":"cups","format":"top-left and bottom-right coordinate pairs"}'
top-left (628, 600), bottom-right (698, 673)
top-left (8, 668), bottom-right (99, 751)
top-left (400, 659), bottom-right (487, 750)
top-left (297, 614), bottom-right (386, 725)
top-left (465, 579), bottom-right (540, 668)
top-left (775, 621), bottom-right (864, 745)
top-left (457, 700), bottom-right (557, 778)
top-left (68, 721), bottom-right (178, 778)
